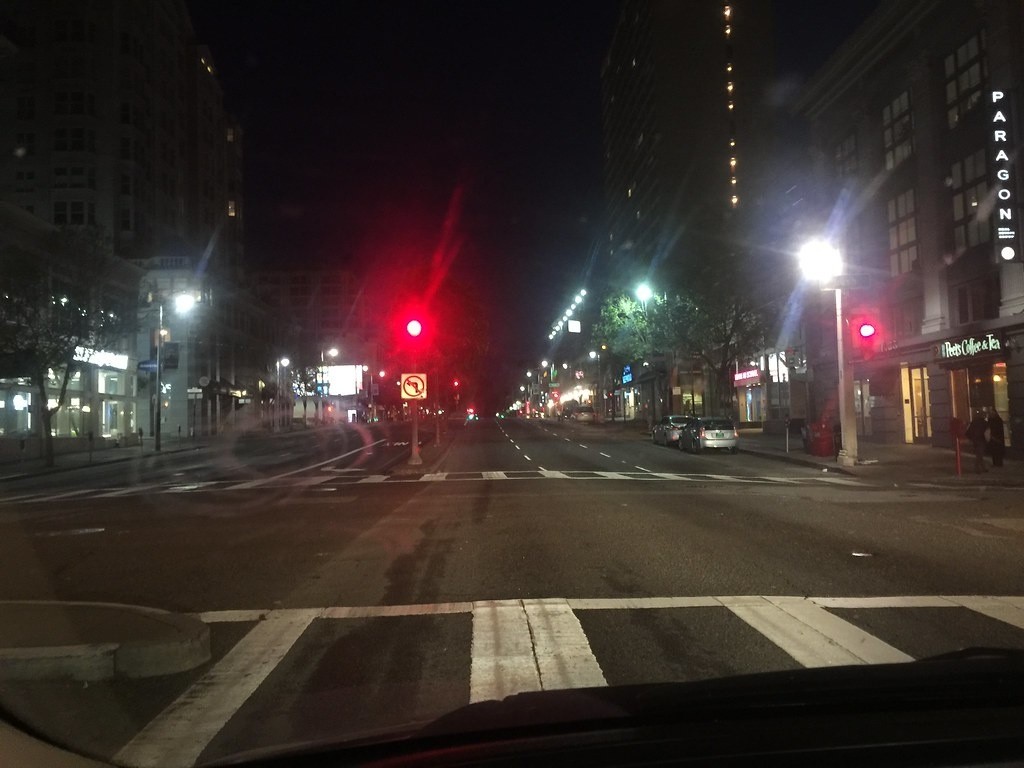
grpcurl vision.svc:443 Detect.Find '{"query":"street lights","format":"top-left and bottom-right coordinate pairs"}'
top-left (370, 367), bottom-right (386, 424)
top-left (354, 361), bottom-right (367, 422)
top-left (275, 353), bottom-right (290, 432)
top-left (802, 244), bottom-right (860, 463)
top-left (155, 285), bottom-right (194, 453)
top-left (320, 346), bottom-right (338, 424)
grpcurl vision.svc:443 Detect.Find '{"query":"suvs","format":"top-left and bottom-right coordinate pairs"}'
top-left (557, 401), bottom-right (579, 424)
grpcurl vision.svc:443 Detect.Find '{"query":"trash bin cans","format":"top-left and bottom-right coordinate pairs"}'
top-left (806, 422), bottom-right (833, 458)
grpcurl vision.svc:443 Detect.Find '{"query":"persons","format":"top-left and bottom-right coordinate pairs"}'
top-left (965, 410), bottom-right (989, 473)
top-left (987, 408), bottom-right (1005, 467)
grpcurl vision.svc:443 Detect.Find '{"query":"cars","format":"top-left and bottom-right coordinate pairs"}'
top-left (679, 416), bottom-right (739, 455)
top-left (651, 415), bottom-right (695, 446)
top-left (570, 405), bottom-right (595, 424)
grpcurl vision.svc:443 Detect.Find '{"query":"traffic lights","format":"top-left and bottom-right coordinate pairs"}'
top-left (404, 316), bottom-right (425, 361)
top-left (454, 381), bottom-right (459, 393)
top-left (859, 317), bottom-right (877, 356)
